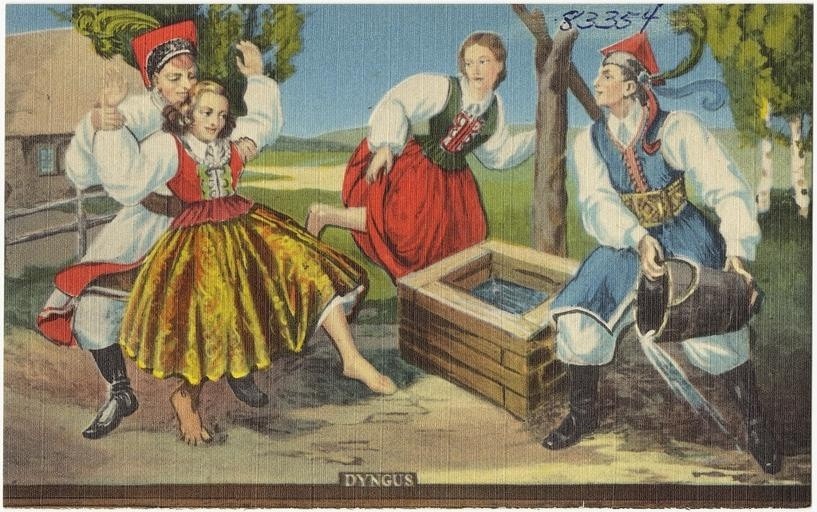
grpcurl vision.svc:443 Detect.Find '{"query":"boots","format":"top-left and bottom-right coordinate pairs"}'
top-left (720, 358), bottom-right (781, 474)
top-left (82, 342), bottom-right (138, 439)
top-left (227, 371), bottom-right (269, 407)
top-left (542, 365), bottom-right (600, 449)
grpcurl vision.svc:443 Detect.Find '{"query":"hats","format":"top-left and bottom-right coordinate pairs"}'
top-left (133, 21), bottom-right (198, 90)
top-left (600, 30), bottom-right (664, 92)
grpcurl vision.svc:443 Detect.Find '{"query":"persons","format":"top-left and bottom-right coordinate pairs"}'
top-left (301, 28), bottom-right (538, 292)
top-left (92, 37), bottom-right (398, 450)
top-left (536, 30), bottom-right (783, 475)
top-left (35, 16), bottom-right (270, 438)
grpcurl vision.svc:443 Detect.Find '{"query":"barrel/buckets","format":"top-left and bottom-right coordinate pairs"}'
top-left (635, 255), bottom-right (751, 340)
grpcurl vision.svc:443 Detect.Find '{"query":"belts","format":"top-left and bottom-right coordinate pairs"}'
top-left (140, 192), bottom-right (186, 219)
